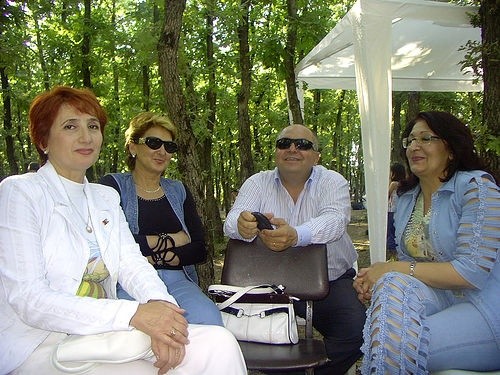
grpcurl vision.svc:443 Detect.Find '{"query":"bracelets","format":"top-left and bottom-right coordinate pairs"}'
top-left (409, 261), bottom-right (416, 277)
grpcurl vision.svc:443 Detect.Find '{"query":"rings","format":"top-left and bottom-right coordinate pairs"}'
top-left (170, 328), bottom-right (176, 337)
top-left (274, 243), bottom-right (275, 247)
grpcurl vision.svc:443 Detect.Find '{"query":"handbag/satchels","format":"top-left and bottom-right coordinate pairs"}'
top-left (50, 329), bottom-right (155, 375)
top-left (208, 282), bottom-right (299, 346)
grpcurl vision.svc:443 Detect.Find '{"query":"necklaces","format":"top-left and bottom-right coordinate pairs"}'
top-left (135, 184), bottom-right (160, 193)
top-left (70, 201), bottom-right (92, 233)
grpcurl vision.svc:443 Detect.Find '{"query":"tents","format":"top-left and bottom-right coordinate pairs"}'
top-left (288, 0), bottom-right (500, 266)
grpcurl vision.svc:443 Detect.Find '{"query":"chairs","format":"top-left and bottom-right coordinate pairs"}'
top-left (221, 239), bottom-right (330, 375)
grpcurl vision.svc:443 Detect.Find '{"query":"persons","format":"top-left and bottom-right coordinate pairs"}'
top-left (353, 111), bottom-right (500, 375)
top-left (388, 163), bottom-right (406, 254)
top-left (224, 124), bottom-right (367, 375)
top-left (0, 86), bottom-right (248, 375)
top-left (98, 108), bottom-right (223, 326)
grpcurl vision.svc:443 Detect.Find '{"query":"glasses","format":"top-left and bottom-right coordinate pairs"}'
top-left (276, 137), bottom-right (314, 151)
top-left (403, 134), bottom-right (441, 148)
top-left (132, 136), bottom-right (178, 153)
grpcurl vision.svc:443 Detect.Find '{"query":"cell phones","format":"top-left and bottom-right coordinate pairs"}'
top-left (251, 212), bottom-right (274, 231)
